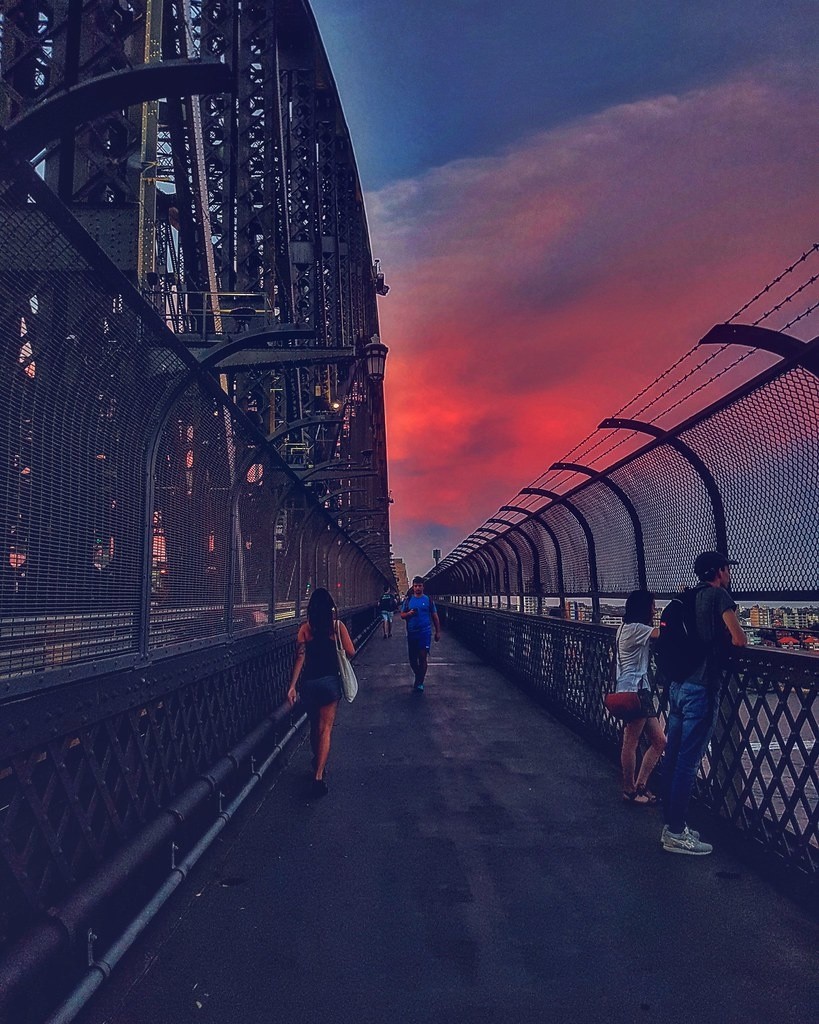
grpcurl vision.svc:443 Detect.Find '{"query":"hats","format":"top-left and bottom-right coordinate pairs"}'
top-left (695, 552), bottom-right (738, 576)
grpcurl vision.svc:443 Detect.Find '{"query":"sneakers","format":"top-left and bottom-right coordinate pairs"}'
top-left (660, 821), bottom-right (713, 856)
top-left (414, 682), bottom-right (424, 694)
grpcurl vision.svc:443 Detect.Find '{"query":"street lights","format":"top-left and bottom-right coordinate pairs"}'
top-left (330, 333), bottom-right (390, 457)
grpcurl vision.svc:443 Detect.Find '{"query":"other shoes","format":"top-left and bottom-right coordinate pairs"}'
top-left (310, 781), bottom-right (328, 797)
top-left (310, 756), bottom-right (328, 779)
top-left (388, 634), bottom-right (392, 638)
top-left (384, 634), bottom-right (387, 639)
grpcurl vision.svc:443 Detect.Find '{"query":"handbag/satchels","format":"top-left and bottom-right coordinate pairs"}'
top-left (333, 619), bottom-right (358, 704)
top-left (605, 693), bottom-right (642, 719)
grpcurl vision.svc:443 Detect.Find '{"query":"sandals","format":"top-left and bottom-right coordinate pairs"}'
top-left (622, 786), bottom-right (659, 808)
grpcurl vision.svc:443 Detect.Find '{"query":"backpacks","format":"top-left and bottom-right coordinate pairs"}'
top-left (660, 585), bottom-right (719, 682)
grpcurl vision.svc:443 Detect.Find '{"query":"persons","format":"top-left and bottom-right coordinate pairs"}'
top-left (660, 551), bottom-right (747, 855)
top-left (394, 591), bottom-right (405, 607)
top-left (401, 576), bottom-right (441, 692)
top-left (377, 586), bottom-right (397, 638)
top-left (287, 588), bottom-right (356, 796)
top-left (613, 590), bottom-right (667, 806)
